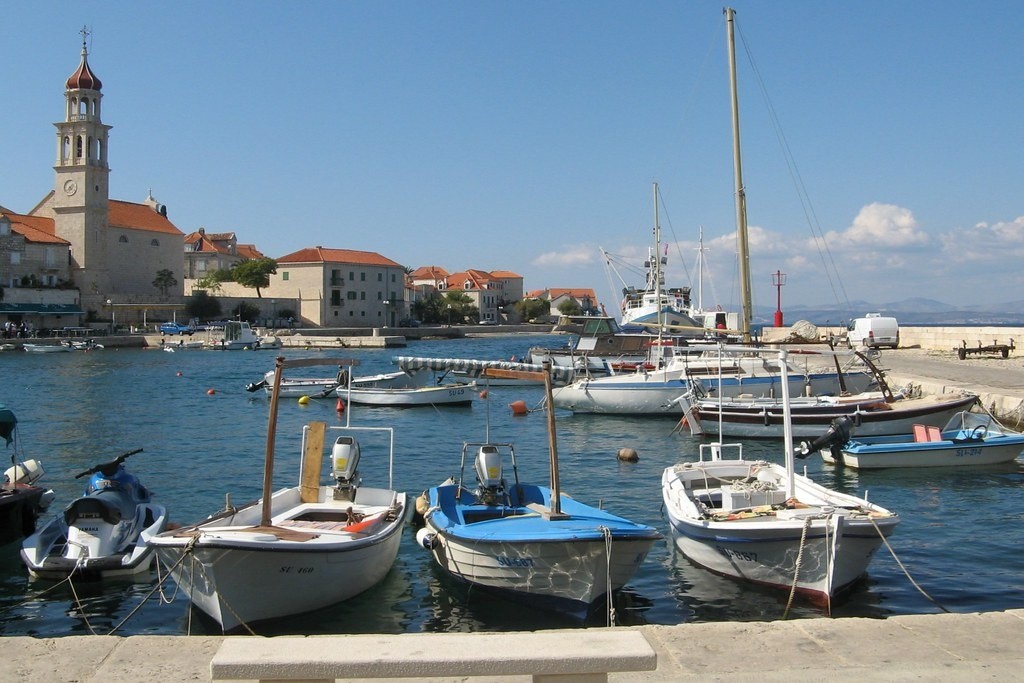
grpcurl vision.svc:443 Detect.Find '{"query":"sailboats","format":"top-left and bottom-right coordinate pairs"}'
top-left (520, 5), bottom-right (893, 416)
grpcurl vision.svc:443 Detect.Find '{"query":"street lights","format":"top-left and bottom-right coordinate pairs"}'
top-left (447, 304), bottom-right (452, 328)
top-left (499, 306), bottom-right (503, 326)
top-left (383, 301), bottom-right (390, 328)
top-left (106, 299), bottom-right (114, 334)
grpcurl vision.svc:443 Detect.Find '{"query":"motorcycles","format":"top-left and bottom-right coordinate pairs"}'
top-left (16, 447), bottom-right (166, 582)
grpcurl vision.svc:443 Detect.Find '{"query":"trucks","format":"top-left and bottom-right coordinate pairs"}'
top-left (847, 313), bottom-right (899, 349)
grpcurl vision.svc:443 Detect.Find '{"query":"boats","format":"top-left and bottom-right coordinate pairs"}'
top-left (660, 360), bottom-right (982, 441)
top-left (794, 410), bottom-right (1024, 474)
top-left (0, 410), bottom-right (56, 544)
top-left (660, 342), bottom-right (902, 612)
top-left (23, 339), bottom-right (75, 352)
top-left (0, 344), bottom-right (16, 351)
top-left (246, 350), bottom-right (407, 399)
top-left (335, 363), bottom-right (480, 407)
top-left (71, 339), bottom-right (104, 351)
top-left (147, 354), bottom-right (412, 630)
top-left (208, 320), bottom-right (259, 350)
top-left (413, 367), bottom-right (667, 604)
top-left (156, 338), bottom-right (204, 348)
top-left (254, 333), bottom-right (284, 350)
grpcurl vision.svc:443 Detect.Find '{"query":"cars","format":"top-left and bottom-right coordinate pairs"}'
top-left (479, 318), bottom-right (498, 325)
top-left (400, 319), bottom-right (422, 328)
top-left (528, 317), bottom-right (546, 324)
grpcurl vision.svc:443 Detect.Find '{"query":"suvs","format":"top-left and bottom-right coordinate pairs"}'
top-left (158, 321), bottom-right (194, 336)
